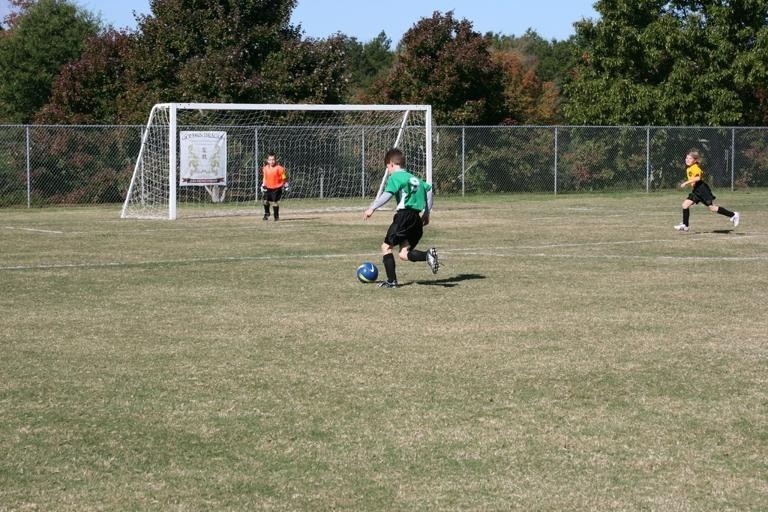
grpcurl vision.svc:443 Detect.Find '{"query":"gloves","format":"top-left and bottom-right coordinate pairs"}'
top-left (281, 186), bottom-right (291, 199)
top-left (260, 185), bottom-right (267, 195)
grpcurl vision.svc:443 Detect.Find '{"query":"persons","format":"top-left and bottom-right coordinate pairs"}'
top-left (363, 149), bottom-right (440, 288)
top-left (260, 152), bottom-right (289, 220)
top-left (675, 150), bottom-right (740, 231)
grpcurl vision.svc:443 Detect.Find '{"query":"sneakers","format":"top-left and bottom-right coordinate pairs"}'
top-left (377, 280), bottom-right (399, 289)
top-left (730, 210), bottom-right (740, 227)
top-left (674, 223), bottom-right (691, 233)
top-left (262, 212), bottom-right (271, 222)
top-left (426, 246), bottom-right (439, 275)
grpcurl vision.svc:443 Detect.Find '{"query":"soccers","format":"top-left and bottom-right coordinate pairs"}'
top-left (356, 262), bottom-right (378, 283)
